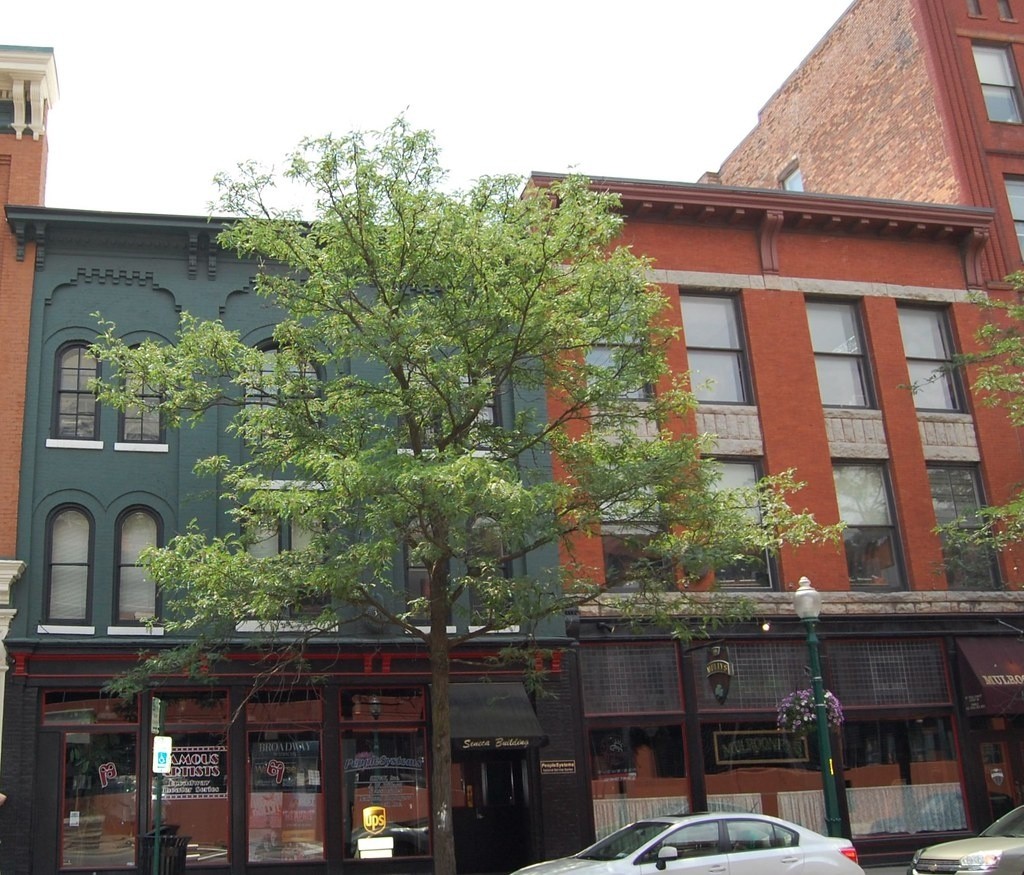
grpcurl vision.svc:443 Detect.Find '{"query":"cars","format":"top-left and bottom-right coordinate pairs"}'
top-left (350, 828), bottom-right (429, 857)
top-left (510, 811), bottom-right (864, 875)
top-left (869, 791), bottom-right (1014, 833)
top-left (596, 799), bottom-right (754, 852)
top-left (906, 805), bottom-right (1024, 875)
top-left (345, 766), bottom-right (425, 788)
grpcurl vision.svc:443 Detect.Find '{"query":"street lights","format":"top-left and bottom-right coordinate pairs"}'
top-left (793, 577), bottom-right (843, 837)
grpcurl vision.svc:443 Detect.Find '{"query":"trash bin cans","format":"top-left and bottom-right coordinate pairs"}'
top-left (136, 835), bottom-right (192, 875)
top-left (151, 824), bottom-right (180, 835)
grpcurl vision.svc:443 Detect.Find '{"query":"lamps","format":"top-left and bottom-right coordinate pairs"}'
top-left (369, 695), bottom-right (382, 720)
top-left (597, 622), bottom-right (615, 634)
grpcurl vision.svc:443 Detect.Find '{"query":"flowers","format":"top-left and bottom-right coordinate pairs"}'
top-left (775, 687), bottom-right (844, 740)
